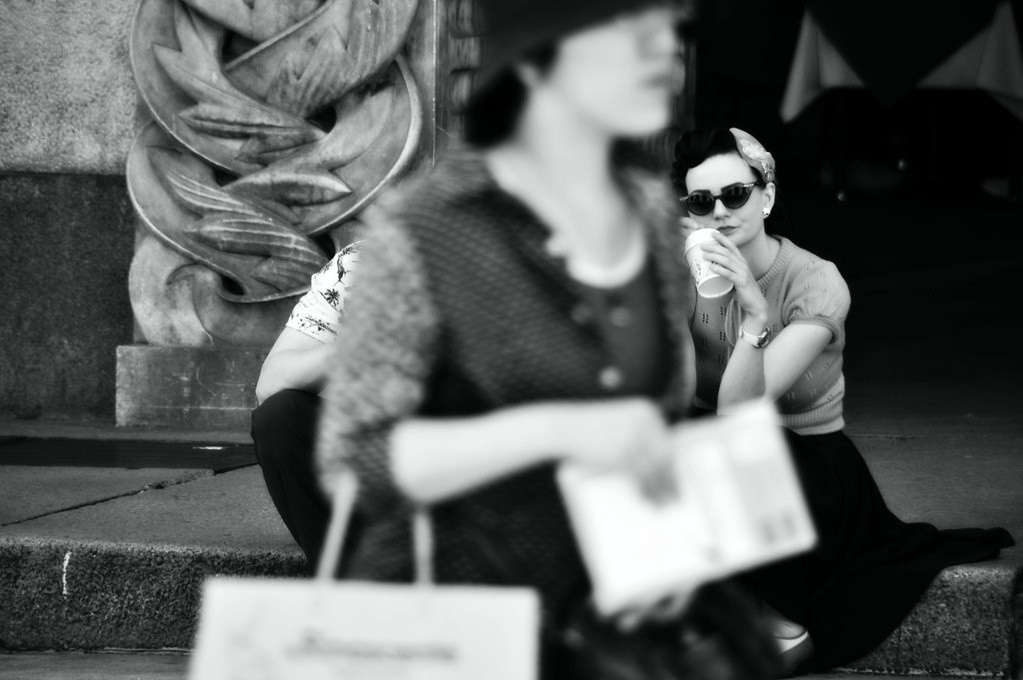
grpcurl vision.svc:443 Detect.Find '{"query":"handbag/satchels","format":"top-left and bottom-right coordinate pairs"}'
top-left (192, 475), bottom-right (540, 680)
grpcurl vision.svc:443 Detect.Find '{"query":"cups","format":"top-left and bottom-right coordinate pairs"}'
top-left (684, 228), bottom-right (734, 300)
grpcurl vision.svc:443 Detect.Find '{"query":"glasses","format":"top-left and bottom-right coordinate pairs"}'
top-left (679, 182), bottom-right (760, 216)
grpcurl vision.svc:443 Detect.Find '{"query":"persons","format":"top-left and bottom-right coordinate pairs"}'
top-left (668, 114), bottom-right (947, 673)
top-left (315, 0), bottom-right (787, 680)
top-left (250, 238), bottom-right (370, 571)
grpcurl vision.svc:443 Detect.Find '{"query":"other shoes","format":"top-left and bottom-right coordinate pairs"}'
top-left (769, 617), bottom-right (813, 676)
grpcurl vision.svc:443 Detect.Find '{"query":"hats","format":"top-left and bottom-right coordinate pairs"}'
top-left (462, 0), bottom-right (693, 146)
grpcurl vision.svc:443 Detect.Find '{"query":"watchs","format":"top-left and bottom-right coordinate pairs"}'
top-left (738, 323), bottom-right (772, 349)
